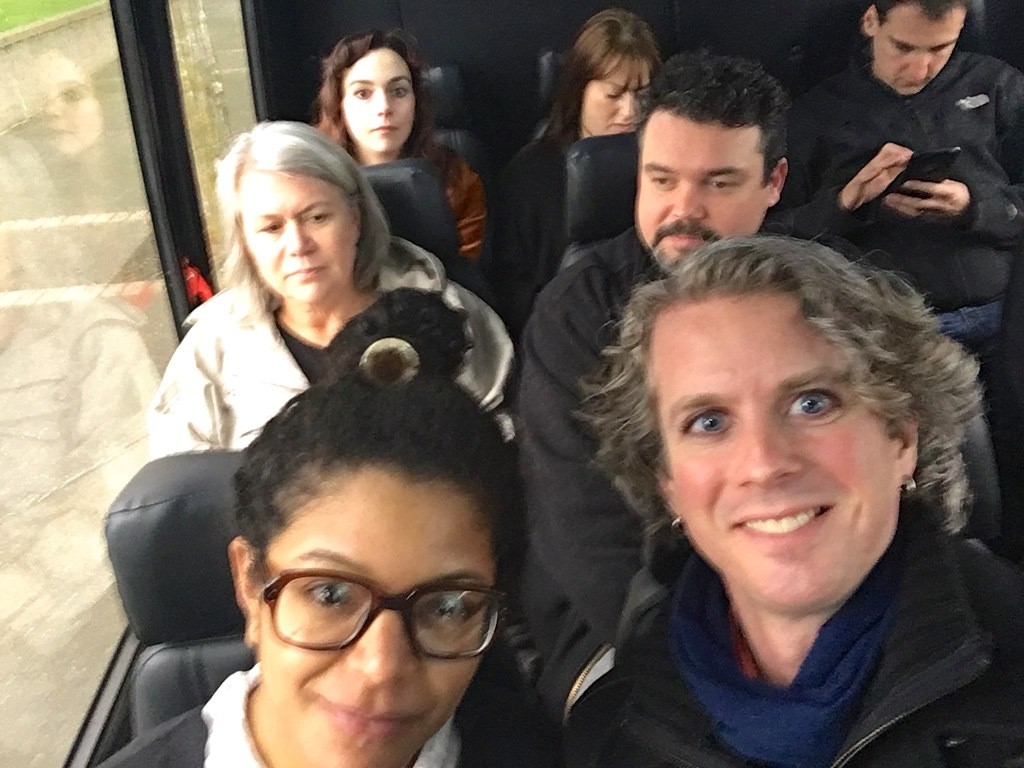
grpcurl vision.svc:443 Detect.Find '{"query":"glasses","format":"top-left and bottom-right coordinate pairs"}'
top-left (249, 552), bottom-right (508, 661)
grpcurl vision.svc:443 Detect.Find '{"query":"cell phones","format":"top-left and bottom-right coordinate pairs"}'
top-left (881, 146), bottom-right (963, 198)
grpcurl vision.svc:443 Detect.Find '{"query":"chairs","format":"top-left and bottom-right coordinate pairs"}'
top-left (526, 44), bottom-right (580, 145)
top-left (557, 131), bottom-right (640, 276)
top-left (362, 157), bottom-right (504, 318)
top-left (407, 62), bottom-right (493, 273)
top-left (101, 449), bottom-right (259, 741)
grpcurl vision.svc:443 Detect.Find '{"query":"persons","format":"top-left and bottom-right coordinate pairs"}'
top-left (2, 42), bottom-right (167, 653)
top-left (94, 0), bottom-right (1024, 768)
